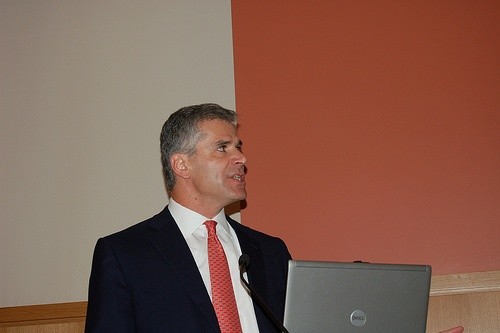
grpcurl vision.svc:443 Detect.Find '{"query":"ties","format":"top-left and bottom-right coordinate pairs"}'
top-left (202, 220), bottom-right (242, 333)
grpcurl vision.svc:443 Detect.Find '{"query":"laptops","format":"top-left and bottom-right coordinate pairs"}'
top-left (283, 260), bottom-right (432, 333)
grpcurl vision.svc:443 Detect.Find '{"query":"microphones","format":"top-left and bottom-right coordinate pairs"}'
top-left (239, 255), bottom-right (289, 333)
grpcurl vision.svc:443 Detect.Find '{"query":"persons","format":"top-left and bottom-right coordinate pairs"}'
top-left (84, 103), bottom-right (293, 333)
